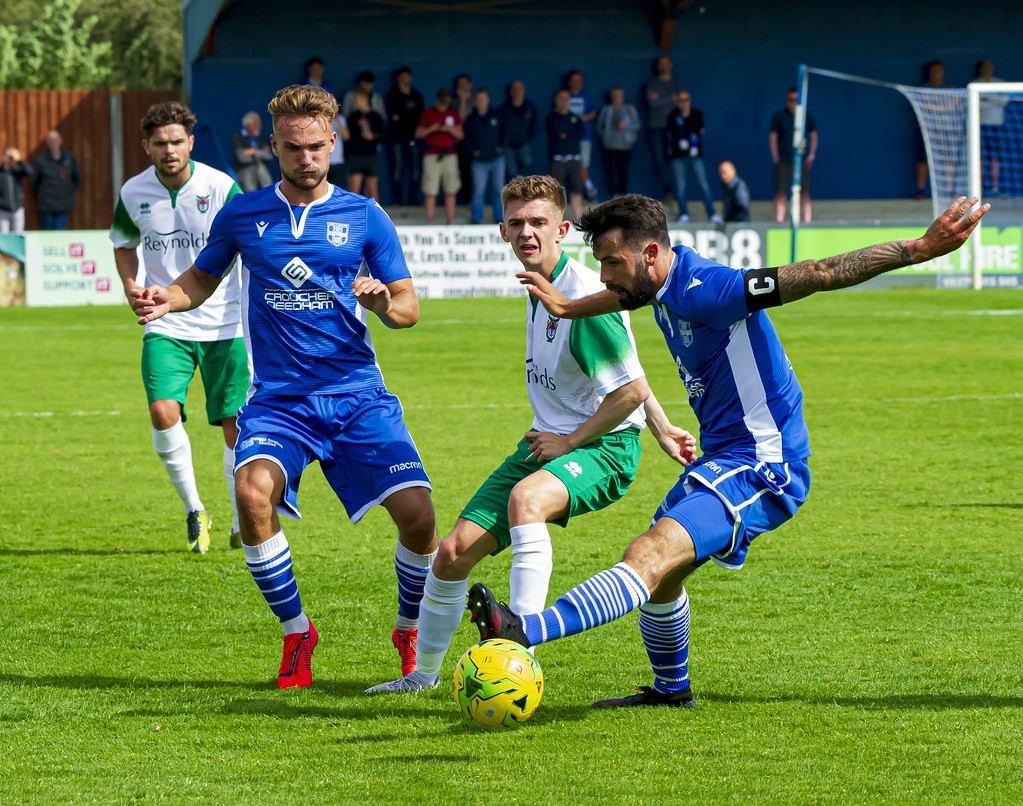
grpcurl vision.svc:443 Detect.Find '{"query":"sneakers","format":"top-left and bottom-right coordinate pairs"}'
top-left (467, 583), bottom-right (532, 649)
top-left (392, 628), bottom-right (418, 677)
top-left (230, 528), bottom-right (243, 549)
top-left (364, 672), bottom-right (440, 695)
top-left (625, 681), bottom-right (693, 708)
top-left (277, 618), bottom-right (319, 690)
top-left (186, 511), bottom-right (212, 554)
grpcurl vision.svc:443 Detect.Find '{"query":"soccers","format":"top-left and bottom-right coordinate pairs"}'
top-left (452, 637), bottom-right (546, 732)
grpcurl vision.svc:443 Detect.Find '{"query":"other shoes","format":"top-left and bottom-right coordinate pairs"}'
top-left (708, 214), bottom-right (725, 226)
top-left (678, 214), bottom-right (690, 224)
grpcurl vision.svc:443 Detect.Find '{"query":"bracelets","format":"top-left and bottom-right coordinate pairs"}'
top-left (809, 152), bottom-right (815, 160)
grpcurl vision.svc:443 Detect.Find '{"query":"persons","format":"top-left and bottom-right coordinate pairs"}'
top-left (466, 196), bottom-right (991, 710)
top-left (916, 60), bottom-right (1009, 201)
top-left (228, 56), bottom-right (818, 226)
top-left (129, 82), bottom-right (440, 688)
top-left (105, 101), bottom-right (250, 557)
top-left (0, 130), bottom-right (83, 233)
top-left (364, 175), bottom-right (697, 692)
